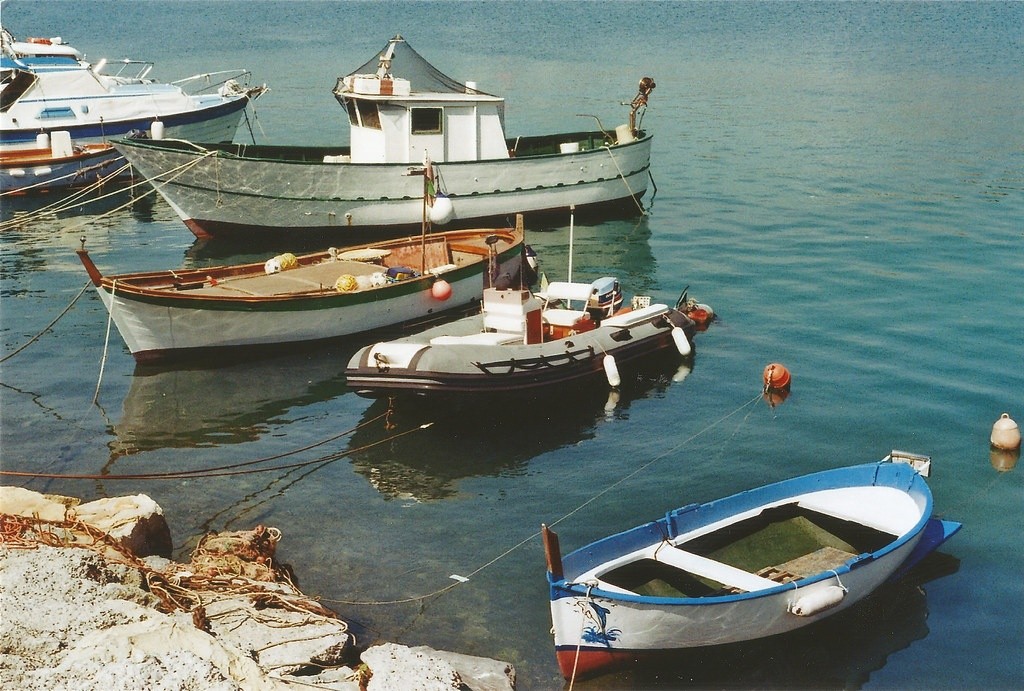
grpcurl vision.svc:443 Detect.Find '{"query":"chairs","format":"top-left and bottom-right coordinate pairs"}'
top-left (541, 281), bottom-right (595, 327)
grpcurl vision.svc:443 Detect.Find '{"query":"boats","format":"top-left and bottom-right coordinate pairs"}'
top-left (343, 203), bottom-right (697, 405)
top-left (541, 437), bottom-right (964, 679)
top-left (75, 213), bottom-right (537, 356)
top-left (0, 23), bottom-right (271, 196)
top-left (109, 33), bottom-right (658, 245)
